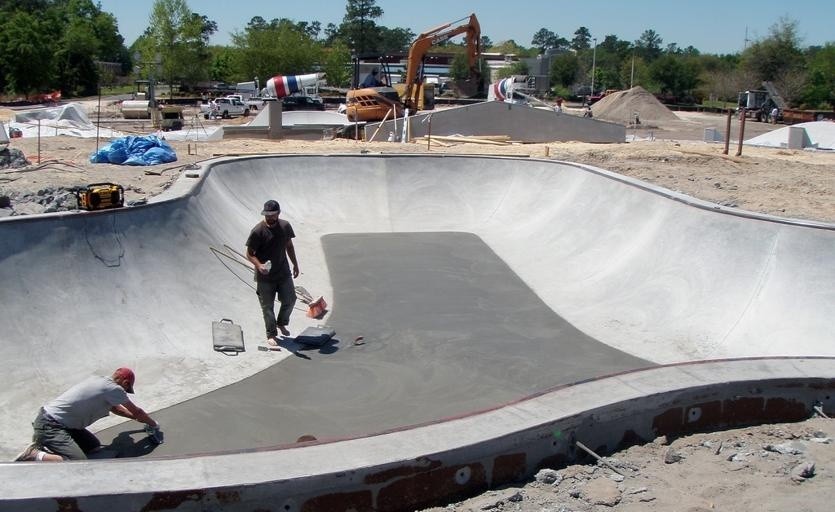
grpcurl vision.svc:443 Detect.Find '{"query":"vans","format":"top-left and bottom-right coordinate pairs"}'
top-left (282, 96), bottom-right (324, 111)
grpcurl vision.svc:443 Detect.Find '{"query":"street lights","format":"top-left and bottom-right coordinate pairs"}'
top-left (628, 44), bottom-right (639, 89)
top-left (590, 38), bottom-right (598, 96)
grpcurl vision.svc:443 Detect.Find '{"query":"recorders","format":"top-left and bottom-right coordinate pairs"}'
top-left (77, 182), bottom-right (125, 211)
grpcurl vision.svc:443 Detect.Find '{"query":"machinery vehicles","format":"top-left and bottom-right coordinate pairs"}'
top-left (487, 73), bottom-right (536, 107)
top-left (345, 13), bottom-right (484, 121)
top-left (121, 80), bottom-right (156, 118)
top-left (149, 97), bottom-right (184, 132)
top-left (587, 89), bottom-right (617, 105)
top-left (236, 72), bottom-right (327, 108)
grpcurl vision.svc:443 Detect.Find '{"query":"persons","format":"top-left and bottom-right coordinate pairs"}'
top-left (771, 106), bottom-right (779, 125)
top-left (337, 103), bottom-right (347, 114)
top-left (556, 96), bottom-right (563, 113)
top-left (245, 199), bottom-right (299, 346)
top-left (584, 108), bottom-right (593, 119)
top-left (10, 367), bottom-right (160, 462)
top-left (207, 99), bottom-right (219, 120)
top-left (360, 67), bottom-right (380, 87)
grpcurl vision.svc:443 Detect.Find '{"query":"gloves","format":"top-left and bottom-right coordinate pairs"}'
top-left (143, 421), bottom-right (160, 435)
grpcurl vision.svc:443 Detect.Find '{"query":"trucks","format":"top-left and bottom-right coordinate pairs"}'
top-left (423, 74), bottom-right (440, 96)
top-left (737, 80), bottom-right (835, 125)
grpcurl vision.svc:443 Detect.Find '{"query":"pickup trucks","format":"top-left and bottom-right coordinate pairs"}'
top-left (226, 95), bottom-right (265, 110)
top-left (199, 97), bottom-right (250, 120)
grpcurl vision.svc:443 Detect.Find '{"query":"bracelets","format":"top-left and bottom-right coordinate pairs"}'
top-left (150, 421), bottom-right (160, 430)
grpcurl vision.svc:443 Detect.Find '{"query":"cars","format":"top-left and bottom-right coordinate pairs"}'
top-left (28, 89), bottom-right (62, 103)
top-left (213, 83), bottom-right (228, 89)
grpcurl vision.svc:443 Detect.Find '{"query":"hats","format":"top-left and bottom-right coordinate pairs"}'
top-left (261, 200), bottom-right (280, 216)
top-left (114, 367), bottom-right (135, 394)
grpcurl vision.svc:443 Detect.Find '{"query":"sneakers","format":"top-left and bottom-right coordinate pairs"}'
top-left (15, 442), bottom-right (38, 461)
